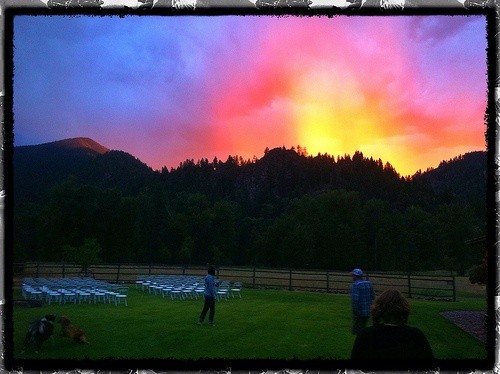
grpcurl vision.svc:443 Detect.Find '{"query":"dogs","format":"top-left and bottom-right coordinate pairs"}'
top-left (23, 313), bottom-right (55, 354)
top-left (57, 321), bottom-right (96, 346)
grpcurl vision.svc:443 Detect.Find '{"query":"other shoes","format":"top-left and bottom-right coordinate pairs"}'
top-left (198, 321), bottom-right (202, 325)
top-left (209, 323), bottom-right (215, 326)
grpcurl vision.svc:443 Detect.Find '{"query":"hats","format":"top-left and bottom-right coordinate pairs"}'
top-left (351, 268), bottom-right (363, 276)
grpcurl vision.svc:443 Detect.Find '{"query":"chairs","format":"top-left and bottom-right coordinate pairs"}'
top-left (22, 278), bottom-right (129, 304)
top-left (136, 274), bottom-right (242, 299)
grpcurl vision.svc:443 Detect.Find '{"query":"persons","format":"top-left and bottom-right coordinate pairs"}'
top-left (349, 269), bottom-right (373, 333)
top-left (350, 289), bottom-right (434, 361)
top-left (198, 267), bottom-right (222, 326)
